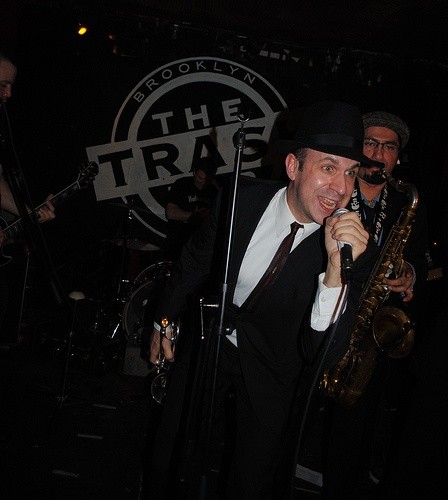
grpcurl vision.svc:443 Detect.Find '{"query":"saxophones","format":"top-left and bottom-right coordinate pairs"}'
top-left (314, 165), bottom-right (421, 411)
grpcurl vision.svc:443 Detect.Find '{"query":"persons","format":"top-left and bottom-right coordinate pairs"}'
top-left (345, 108), bottom-right (428, 376)
top-left (0, 56), bottom-right (56, 242)
top-left (157, 156), bottom-right (222, 272)
top-left (149, 101), bottom-right (369, 500)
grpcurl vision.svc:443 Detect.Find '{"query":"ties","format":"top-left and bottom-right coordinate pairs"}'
top-left (239, 222), bottom-right (304, 311)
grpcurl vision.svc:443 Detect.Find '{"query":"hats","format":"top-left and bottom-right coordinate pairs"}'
top-left (362, 110), bottom-right (410, 153)
top-left (265, 100), bottom-right (385, 168)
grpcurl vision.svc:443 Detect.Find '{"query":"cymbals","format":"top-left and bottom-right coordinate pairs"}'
top-left (107, 202), bottom-right (154, 217)
top-left (103, 236), bottom-right (162, 251)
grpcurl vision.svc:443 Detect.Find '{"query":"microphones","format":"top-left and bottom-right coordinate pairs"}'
top-left (333, 208), bottom-right (354, 272)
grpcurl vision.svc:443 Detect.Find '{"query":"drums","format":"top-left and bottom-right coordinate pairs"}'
top-left (121, 259), bottom-right (176, 346)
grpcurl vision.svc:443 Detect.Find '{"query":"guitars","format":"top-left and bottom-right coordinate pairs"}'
top-left (0, 159), bottom-right (100, 266)
